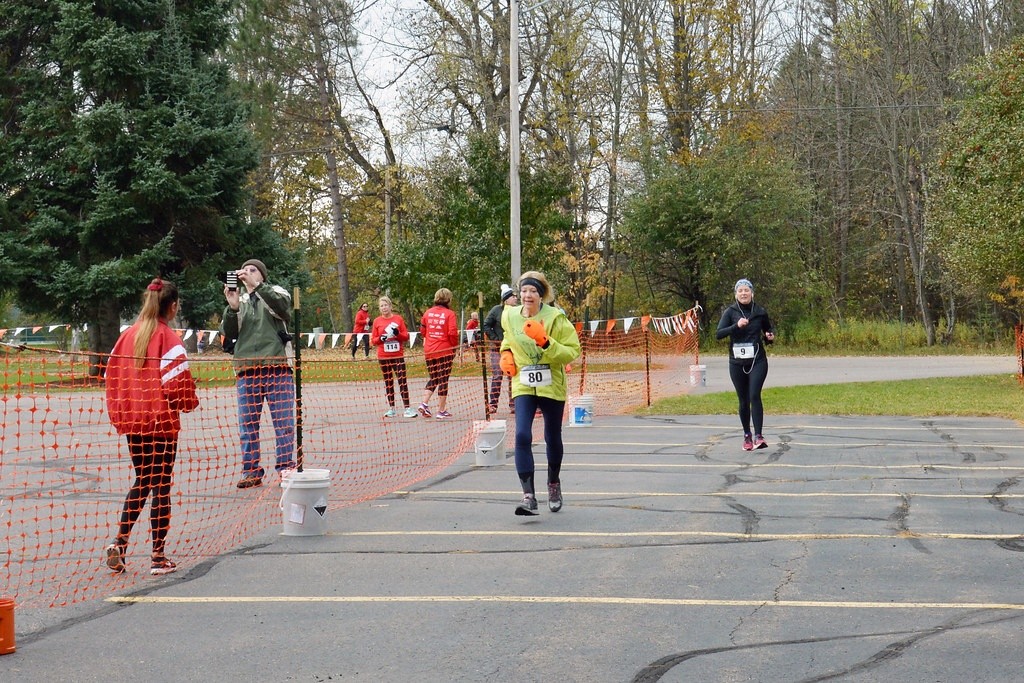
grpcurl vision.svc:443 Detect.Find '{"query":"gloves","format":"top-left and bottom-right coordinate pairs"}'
top-left (499, 350), bottom-right (516, 376)
top-left (391, 328), bottom-right (399, 335)
top-left (381, 335), bottom-right (387, 342)
top-left (523, 319), bottom-right (548, 348)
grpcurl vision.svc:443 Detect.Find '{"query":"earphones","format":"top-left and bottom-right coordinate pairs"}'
top-left (735, 295), bottom-right (738, 300)
top-left (752, 293), bottom-right (754, 298)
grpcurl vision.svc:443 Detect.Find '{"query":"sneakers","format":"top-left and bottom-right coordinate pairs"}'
top-left (106, 540), bottom-right (126, 573)
top-left (515, 493), bottom-right (540, 515)
top-left (150, 554), bottom-right (177, 574)
top-left (742, 431), bottom-right (754, 451)
top-left (418, 403), bottom-right (432, 417)
top-left (753, 434), bottom-right (768, 449)
top-left (547, 477), bottom-right (563, 511)
top-left (436, 410), bottom-right (452, 418)
top-left (403, 408), bottom-right (418, 418)
top-left (384, 409), bottom-right (397, 417)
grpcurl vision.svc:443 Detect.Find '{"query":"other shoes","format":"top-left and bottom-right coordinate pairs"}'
top-left (237, 477), bottom-right (262, 488)
top-left (365, 357), bottom-right (372, 361)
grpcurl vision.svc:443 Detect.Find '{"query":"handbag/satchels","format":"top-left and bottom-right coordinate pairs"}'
top-left (351, 356), bottom-right (355, 361)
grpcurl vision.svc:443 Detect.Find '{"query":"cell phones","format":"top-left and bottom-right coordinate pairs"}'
top-left (227, 271), bottom-right (237, 291)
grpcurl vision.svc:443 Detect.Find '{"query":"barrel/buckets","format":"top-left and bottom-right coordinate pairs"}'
top-left (473, 420), bottom-right (507, 467)
top-left (569, 394), bottom-right (595, 427)
top-left (280, 469), bottom-right (330, 535)
top-left (689, 365), bottom-right (707, 388)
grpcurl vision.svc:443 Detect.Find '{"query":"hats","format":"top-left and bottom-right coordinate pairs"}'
top-left (241, 259), bottom-right (267, 282)
top-left (500, 284), bottom-right (517, 302)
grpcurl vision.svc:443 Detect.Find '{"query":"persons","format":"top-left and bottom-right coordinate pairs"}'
top-left (372, 296), bottom-right (418, 418)
top-left (418, 287), bottom-right (459, 419)
top-left (351, 302), bottom-right (372, 360)
top-left (466, 312), bottom-right (479, 362)
top-left (499, 271), bottom-right (581, 516)
top-left (484, 283), bottom-right (517, 413)
top-left (219, 258), bottom-right (296, 488)
top-left (717, 279), bottom-right (775, 451)
top-left (106, 277), bottom-right (199, 574)
